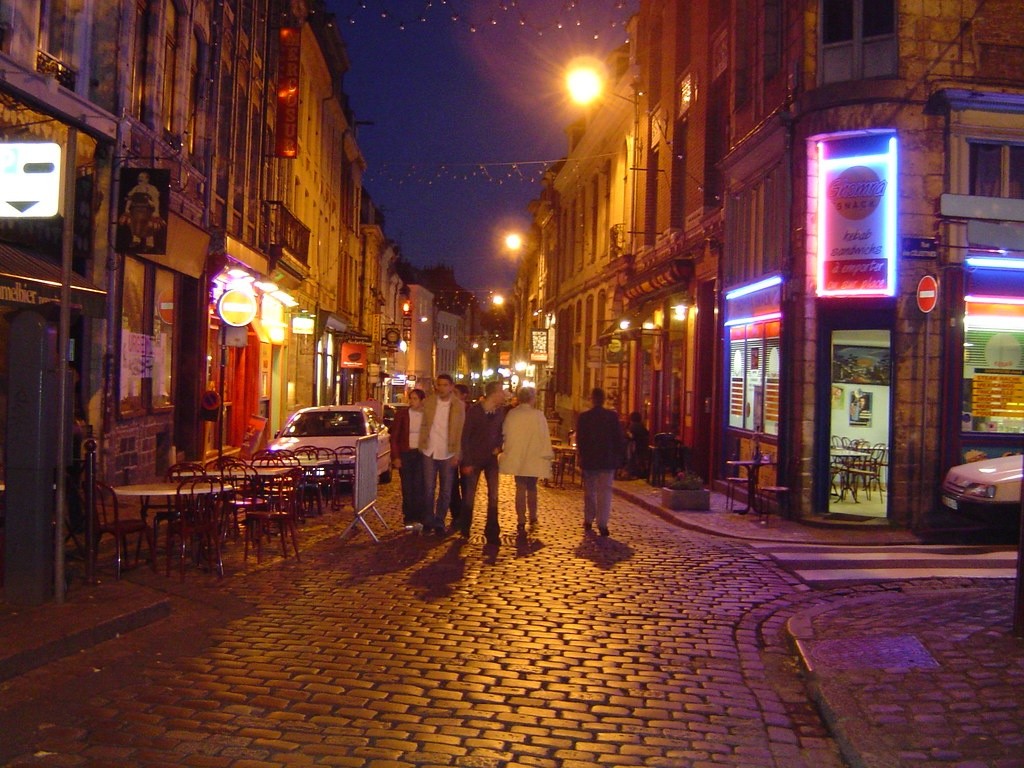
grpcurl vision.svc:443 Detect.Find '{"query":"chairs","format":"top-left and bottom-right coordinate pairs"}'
top-left (830, 435), bottom-right (887, 503)
top-left (81, 446), bottom-right (356, 584)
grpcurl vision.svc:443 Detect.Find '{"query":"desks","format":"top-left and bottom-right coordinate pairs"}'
top-left (726, 460), bottom-right (770, 516)
top-left (236, 459), bottom-right (331, 515)
top-left (545, 446), bottom-right (581, 489)
top-left (111, 484), bottom-right (240, 567)
top-left (831, 448), bottom-right (871, 503)
top-left (171, 468), bottom-right (292, 539)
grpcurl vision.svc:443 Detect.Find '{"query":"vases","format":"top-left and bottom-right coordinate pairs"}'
top-left (661, 488), bottom-right (712, 511)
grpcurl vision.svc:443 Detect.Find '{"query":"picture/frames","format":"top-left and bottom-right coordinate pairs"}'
top-left (832, 343), bottom-right (890, 387)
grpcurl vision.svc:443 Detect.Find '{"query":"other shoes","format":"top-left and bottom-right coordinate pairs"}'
top-left (423, 522), bottom-right (432, 532)
top-left (530, 518), bottom-right (539, 525)
top-left (517, 523), bottom-right (525, 531)
top-left (460, 522), bottom-right (470, 539)
top-left (484, 529), bottom-right (501, 544)
top-left (583, 522), bottom-right (592, 531)
top-left (433, 520), bottom-right (448, 537)
top-left (597, 523), bottom-right (610, 536)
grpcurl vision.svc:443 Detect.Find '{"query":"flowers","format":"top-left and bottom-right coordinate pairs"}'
top-left (665, 469), bottom-right (703, 490)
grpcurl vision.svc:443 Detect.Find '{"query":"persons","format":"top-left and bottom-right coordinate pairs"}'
top-left (497, 387), bottom-right (556, 533)
top-left (625, 411), bottom-right (650, 479)
top-left (388, 388), bottom-right (425, 528)
top-left (856, 397), bottom-right (869, 422)
top-left (457, 383), bottom-right (509, 544)
top-left (505, 396), bottom-right (519, 409)
top-left (452, 383), bottom-right (485, 414)
top-left (577, 386), bottom-right (626, 536)
top-left (416, 373), bottom-right (467, 536)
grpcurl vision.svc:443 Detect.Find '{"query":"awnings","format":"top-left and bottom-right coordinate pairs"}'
top-left (326, 330), bottom-right (373, 347)
top-left (0, 241), bottom-right (109, 319)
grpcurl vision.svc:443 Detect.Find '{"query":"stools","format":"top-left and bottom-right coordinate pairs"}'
top-left (727, 478), bottom-right (747, 512)
top-left (759, 486), bottom-right (787, 527)
